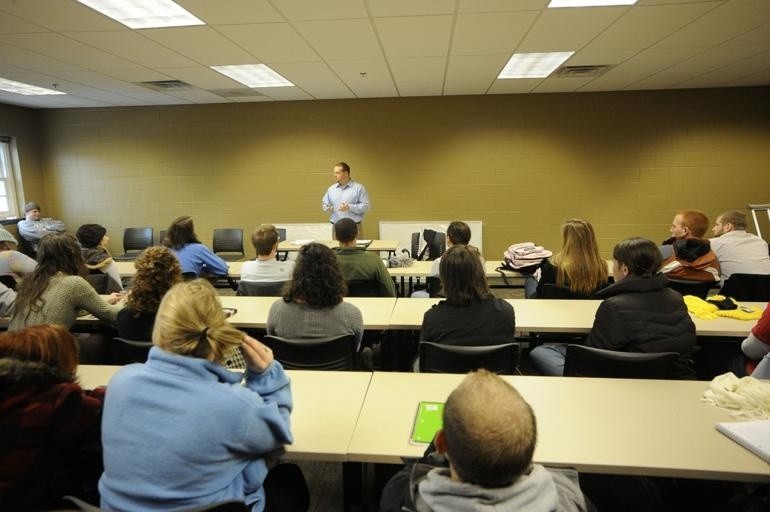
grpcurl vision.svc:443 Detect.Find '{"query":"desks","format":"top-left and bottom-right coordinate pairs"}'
top-left (72, 293), bottom-right (396, 348)
top-left (74, 362), bottom-right (372, 469)
top-left (117, 261), bottom-right (404, 280)
top-left (347, 372), bottom-right (769, 483)
top-left (276, 238), bottom-right (399, 267)
top-left (111, 337), bottom-right (152, 362)
top-left (387, 294), bottom-right (769, 338)
top-left (404, 256), bottom-right (614, 284)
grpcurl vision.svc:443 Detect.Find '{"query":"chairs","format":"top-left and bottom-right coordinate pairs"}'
top-left (537, 284), bottom-right (574, 298)
top-left (400, 232), bottom-right (445, 293)
top-left (668, 277), bottom-right (712, 301)
top-left (424, 276), bottom-right (444, 298)
top-left (113, 227), bottom-right (153, 262)
top-left (717, 273), bottom-right (770, 302)
top-left (62, 494), bottom-right (247, 512)
top-left (418, 341), bottom-right (520, 376)
top-left (263, 333), bottom-right (358, 370)
top-left (211, 228), bottom-right (245, 262)
top-left (159, 230), bottom-right (167, 247)
top-left (562, 343), bottom-right (680, 378)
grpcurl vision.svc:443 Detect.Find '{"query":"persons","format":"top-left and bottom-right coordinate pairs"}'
top-left (97, 279), bottom-right (311, 512)
top-left (524, 217), bottom-right (609, 299)
top-left (167, 214), bottom-right (230, 280)
top-left (411, 221), bottom-right (473, 299)
top-left (406, 243), bottom-right (517, 373)
top-left (321, 162), bottom-right (369, 240)
top-left (76, 222), bottom-right (124, 294)
top-left (379, 370), bottom-right (594, 512)
top-left (330, 218), bottom-right (398, 298)
top-left (266, 242), bottom-right (374, 372)
top-left (656, 210), bottom-right (722, 287)
top-left (0, 322), bottom-right (107, 512)
top-left (741, 300), bottom-right (770, 379)
top-left (708, 209), bottom-right (770, 287)
top-left (0, 227), bottom-right (37, 316)
top-left (16, 201), bottom-right (67, 253)
top-left (529, 236), bottom-right (697, 376)
top-left (8, 231), bottom-right (131, 366)
top-left (117, 245), bottom-right (185, 340)
top-left (241, 224), bottom-right (295, 282)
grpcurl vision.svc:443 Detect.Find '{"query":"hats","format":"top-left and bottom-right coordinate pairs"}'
top-left (0, 227), bottom-right (18, 244)
top-left (25, 200), bottom-right (39, 211)
top-left (75, 223), bottom-right (106, 248)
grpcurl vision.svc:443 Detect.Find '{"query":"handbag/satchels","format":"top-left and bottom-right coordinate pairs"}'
top-left (494, 242), bottom-right (548, 286)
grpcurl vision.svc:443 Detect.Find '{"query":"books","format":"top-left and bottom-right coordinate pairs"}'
top-left (410, 400), bottom-right (444, 447)
